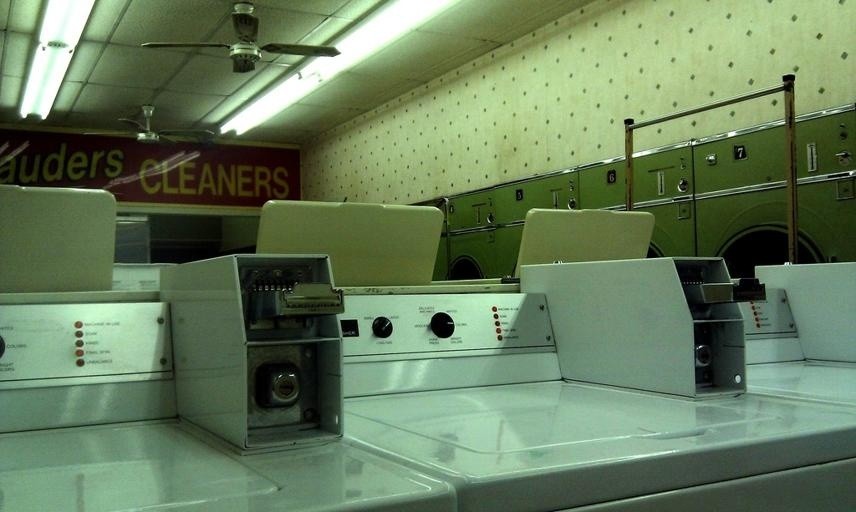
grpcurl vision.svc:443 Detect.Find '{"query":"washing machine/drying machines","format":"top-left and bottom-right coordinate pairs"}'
top-left (0, 100), bottom-right (856, 512)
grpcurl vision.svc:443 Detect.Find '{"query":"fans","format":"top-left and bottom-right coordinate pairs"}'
top-left (136, 2), bottom-right (341, 74)
top-left (80, 106), bottom-right (215, 144)
